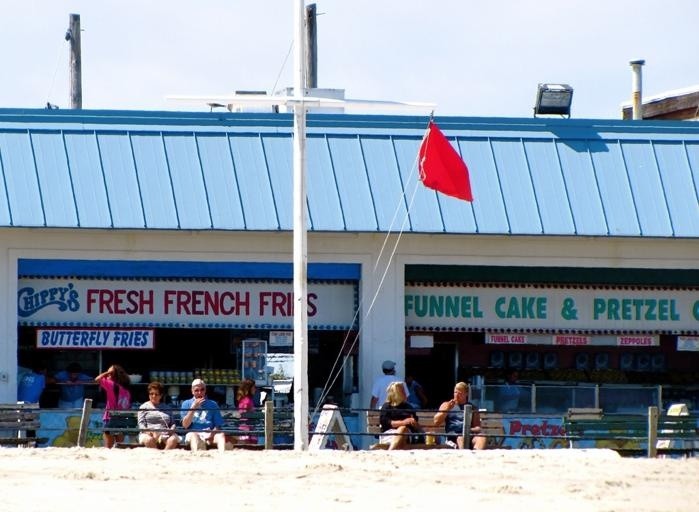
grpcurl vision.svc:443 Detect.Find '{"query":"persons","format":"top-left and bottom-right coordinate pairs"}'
top-left (137, 380), bottom-right (178, 449)
top-left (94, 363), bottom-right (131, 448)
top-left (376, 382), bottom-right (422, 451)
top-left (369, 359), bottom-right (411, 409)
top-left (404, 371), bottom-right (428, 409)
top-left (44, 362), bottom-right (96, 408)
top-left (17, 364), bottom-right (56, 410)
top-left (237, 377), bottom-right (258, 444)
top-left (179, 378), bottom-right (226, 450)
top-left (432, 381), bottom-right (487, 449)
top-left (494, 366), bottom-right (523, 411)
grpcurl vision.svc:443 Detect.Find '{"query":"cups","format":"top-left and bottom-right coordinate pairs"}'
top-left (425, 432), bottom-right (433, 446)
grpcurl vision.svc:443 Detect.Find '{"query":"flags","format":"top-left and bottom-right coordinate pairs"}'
top-left (416, 119), bottom-right (475, 206)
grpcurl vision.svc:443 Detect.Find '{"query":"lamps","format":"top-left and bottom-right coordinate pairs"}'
top-left (533, 82), bottom-right (573, 120)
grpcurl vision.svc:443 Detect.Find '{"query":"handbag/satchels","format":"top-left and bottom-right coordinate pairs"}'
top-left (110, 416), bottom-right (140, 436)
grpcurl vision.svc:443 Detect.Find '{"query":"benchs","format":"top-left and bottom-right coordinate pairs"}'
top-left (365, 408), bottom-right (512, 450)
top-left (104, 410), bottom-right (295, 448)
top-left (563, 414), bottom-right (699, 457)
top-left (0, 401), bottom-right (50, 448)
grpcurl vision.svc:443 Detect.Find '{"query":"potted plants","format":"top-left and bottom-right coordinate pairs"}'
top-left (270, 373), bottom-right (294, 393)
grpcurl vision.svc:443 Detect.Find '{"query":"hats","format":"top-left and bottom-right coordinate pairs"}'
top-left (382, 361), bottom-right (396, 369)
